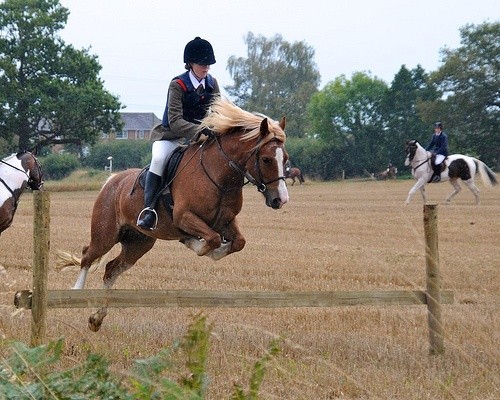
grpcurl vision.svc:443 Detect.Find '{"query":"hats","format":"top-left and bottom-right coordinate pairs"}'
top-left (184, 37), bottom-right (216, 66)
top-left (433, 122), bottom-right (442, 129)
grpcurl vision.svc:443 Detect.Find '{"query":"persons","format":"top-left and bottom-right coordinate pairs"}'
top-left (386, 160), bottom-right (392, 172)
top-left (285, 159), bottom-right (290, 176)
top-left (138, 37), bottom-right (221, 231)
top-left (426, 122), bottom-right (448, 181)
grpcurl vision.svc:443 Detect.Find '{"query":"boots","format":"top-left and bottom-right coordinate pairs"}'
top-left (433, 165), bottom-right (440, 182)
top-left (137, 171), bottom-right (162, 230)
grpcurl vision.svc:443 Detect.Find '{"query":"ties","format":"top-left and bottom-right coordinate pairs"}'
top-left (197, 84), bottom-right (203, 94)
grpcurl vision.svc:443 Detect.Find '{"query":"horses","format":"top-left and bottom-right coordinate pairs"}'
top-left (374, 167), bottom-right (397, 181)
top-left (403, 139), bottom-right (500, 204)
top-left (56, 98), bottom-right (288, 332)
top-left (285, 168), bottom-right (305, 185)
top-left (1, 150), bottom-right (43, 238)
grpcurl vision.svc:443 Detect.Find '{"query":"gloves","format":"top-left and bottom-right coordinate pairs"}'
top-left (432, 153), bottom-right (436, 158)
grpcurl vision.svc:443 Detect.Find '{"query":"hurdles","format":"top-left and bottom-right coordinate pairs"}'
top-left (14, 189), bottom-right (455, 355)
